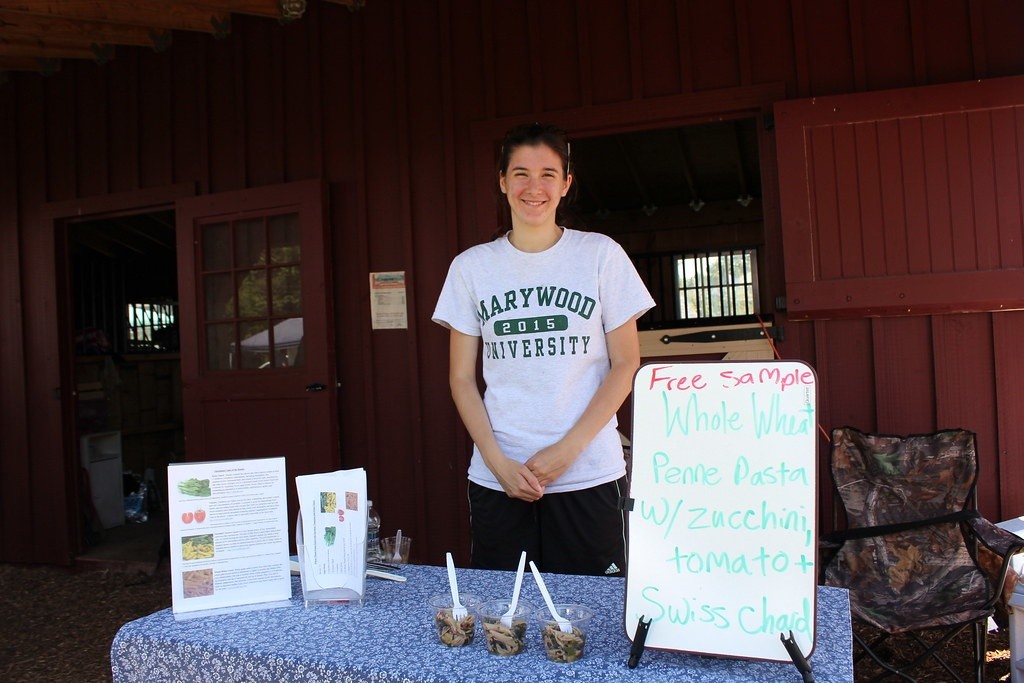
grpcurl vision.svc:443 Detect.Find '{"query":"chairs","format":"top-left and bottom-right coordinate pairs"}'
top-left (820, 425), bottom-right (1024, 683)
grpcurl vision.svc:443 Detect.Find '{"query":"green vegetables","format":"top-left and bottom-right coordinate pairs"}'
top-left (436, 618), bottom-right (474, 645)
top-left (542, 625), bottom-right (581, 656)
top-left (487, 619), bottom-right (526, 653)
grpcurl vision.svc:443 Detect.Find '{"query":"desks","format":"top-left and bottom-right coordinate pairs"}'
top-left (108, 560), bottom-right (856, 683)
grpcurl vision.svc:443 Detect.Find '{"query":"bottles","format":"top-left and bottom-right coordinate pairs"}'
top-left (367, 501), bottom-right (381, 566)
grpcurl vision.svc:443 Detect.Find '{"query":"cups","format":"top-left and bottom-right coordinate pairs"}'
top-left (538, 605), bottom-right (596, 662)
top-left (428, 593), bottom-right (479, 647)
top-left (381, 537), bottom-right (411, 564)
top-left (478, 600), bottom-right (533, 657)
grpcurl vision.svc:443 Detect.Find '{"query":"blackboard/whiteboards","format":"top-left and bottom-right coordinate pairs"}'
top-left (620, 358), bottom-right (821, 660)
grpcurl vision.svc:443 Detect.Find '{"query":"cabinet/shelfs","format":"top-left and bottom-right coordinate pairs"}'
top-left (73, 354), bottom-right (183, 527)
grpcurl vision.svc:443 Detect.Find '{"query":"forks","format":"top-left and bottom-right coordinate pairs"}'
top-left (392, 530), bottom-right (403, 566)
top-left (445, 551), bottom-right (469, 621)
top-left (500, 551), bottom-right (527, 628)
top-left (529, 561), bottom-right (572, 635)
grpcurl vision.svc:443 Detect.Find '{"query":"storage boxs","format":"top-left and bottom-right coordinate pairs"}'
top-left (988, 515), bottom-right (1024, 683)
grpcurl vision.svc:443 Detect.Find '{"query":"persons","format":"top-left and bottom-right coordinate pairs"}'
top-left (113, 364), bottom-right (138, 412)
top-left (430, 123), bottom-right (656, 577)
